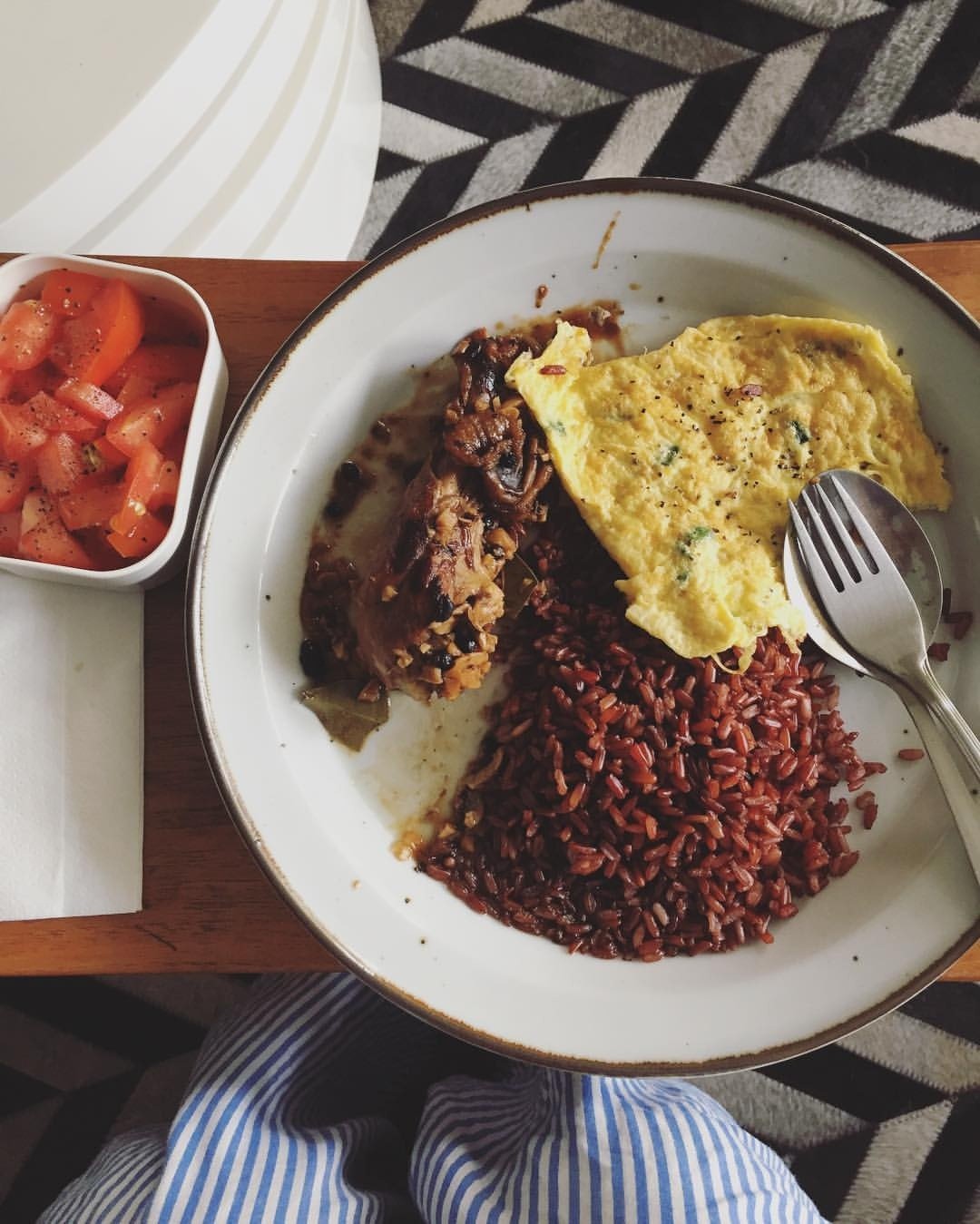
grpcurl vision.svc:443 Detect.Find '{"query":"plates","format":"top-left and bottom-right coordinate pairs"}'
top-left (185, 174), bottom-right (980, 1077)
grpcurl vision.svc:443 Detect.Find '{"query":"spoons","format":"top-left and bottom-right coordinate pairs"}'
top-left (779, 470), bottom-right (980, 886)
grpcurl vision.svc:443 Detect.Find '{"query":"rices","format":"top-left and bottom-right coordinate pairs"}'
top-left (427, 520), bottom-right (972, 960)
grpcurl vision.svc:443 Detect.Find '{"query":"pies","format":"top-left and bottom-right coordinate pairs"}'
top-left (511, 307), bottom-right (954, 664)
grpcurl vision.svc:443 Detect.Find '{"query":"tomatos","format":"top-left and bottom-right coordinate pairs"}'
top-left (1, 270), bottom-right (203, 570)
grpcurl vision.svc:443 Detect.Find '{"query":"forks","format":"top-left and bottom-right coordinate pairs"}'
top-left (792, 473), bottom-right (979, 788)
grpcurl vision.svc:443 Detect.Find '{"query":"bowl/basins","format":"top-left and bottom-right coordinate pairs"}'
top-left (0, 253), bottom-right (228, 591)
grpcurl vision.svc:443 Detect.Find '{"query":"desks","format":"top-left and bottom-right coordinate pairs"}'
top-left (4, 235), bottom-right (974, 1004)
